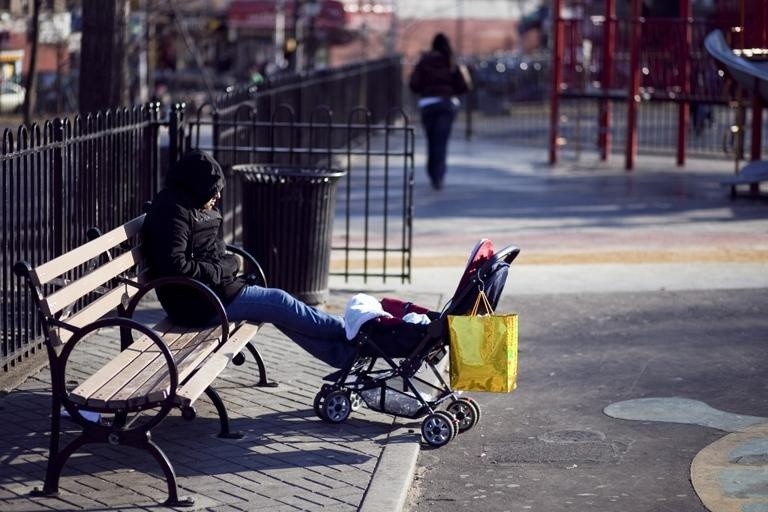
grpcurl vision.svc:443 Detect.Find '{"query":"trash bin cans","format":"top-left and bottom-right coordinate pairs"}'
top-left (231, 164), bottom-right (348, 306)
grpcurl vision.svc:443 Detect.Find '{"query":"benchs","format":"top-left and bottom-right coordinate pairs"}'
top-left (12, 200), bottom-right (279, 507)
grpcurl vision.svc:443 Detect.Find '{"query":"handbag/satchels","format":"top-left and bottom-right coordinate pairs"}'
top-left (447, 292), bottom-right (519, 394)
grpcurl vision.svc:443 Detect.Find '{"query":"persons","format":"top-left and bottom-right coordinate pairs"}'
top-left (137, 147), bottom-right (372, 372)
top-left (406, 32), bottom-right (475, 189)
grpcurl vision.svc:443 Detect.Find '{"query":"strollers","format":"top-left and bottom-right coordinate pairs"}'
top-left (312, 238), bottom-right (519, 445)
